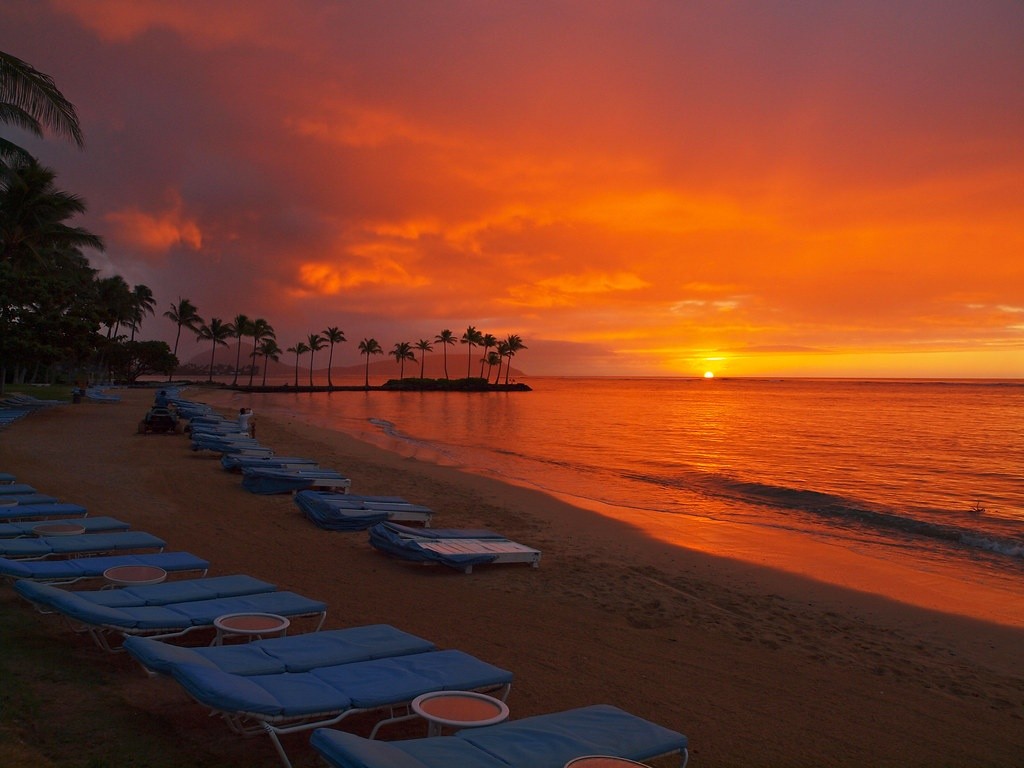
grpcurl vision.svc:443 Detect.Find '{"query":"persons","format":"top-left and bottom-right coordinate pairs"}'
top-left (154, 390), bottom-right (167, 411)
top-left (238, 407), bottom-right (254, 436)
top-left (71, 383), bottom-right (81, 401)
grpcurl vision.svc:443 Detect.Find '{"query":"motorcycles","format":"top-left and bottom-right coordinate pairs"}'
top-left (138, 405), bottom-right (184, 434)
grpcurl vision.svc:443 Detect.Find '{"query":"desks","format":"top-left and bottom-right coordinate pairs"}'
top-left (213, 612), bottom-right (290, 645)
top-left (0, 498), bottom-right (19, 507)
top-left (563, 755), bottom-right (653, 768)
top-left (33, 525), bottom-right (85, 538)
top-left (102, 565), bottom-right (166, 590)
top-left (413, 690), bottom-right (510, 738)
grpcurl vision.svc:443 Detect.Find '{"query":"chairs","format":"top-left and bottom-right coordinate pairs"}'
top-left (0, 384), bottom-right (690, 768)
